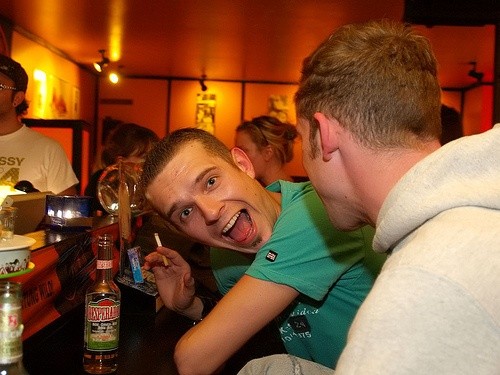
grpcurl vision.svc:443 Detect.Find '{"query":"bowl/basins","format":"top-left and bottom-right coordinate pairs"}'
top-left (0, 235), bottom-right (36, 275)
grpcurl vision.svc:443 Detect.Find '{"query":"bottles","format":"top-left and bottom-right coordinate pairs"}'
top-left (83, 234), bottom-right (121, 374)
top-left (0, 281), bottom-right (30, 375)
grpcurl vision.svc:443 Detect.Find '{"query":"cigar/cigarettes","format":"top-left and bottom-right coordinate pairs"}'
top-left (154, 233), bottom-right (168, 266)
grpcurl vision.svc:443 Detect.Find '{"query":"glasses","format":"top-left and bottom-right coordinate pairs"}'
top-left (0, 85), bottom-right (17, 91)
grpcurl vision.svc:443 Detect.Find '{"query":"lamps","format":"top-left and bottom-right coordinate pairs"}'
top-left (199, 80), bottom-right (208, 91)
top-left (93, 58), bottom-right (110, 72)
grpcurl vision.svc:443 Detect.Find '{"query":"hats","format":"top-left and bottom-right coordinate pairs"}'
top-left (0, 54), bottom-right (28, 91)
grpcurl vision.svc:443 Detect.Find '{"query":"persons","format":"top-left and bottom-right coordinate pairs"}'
top-left (0, 54), bottom-right (79, 195)
top-left (234, 116), bottom-right (310, 188)
top-left (237, 18), bottom-right (500, 375)
top-left (142, 127), bottom-right (387, 375)
top-left (84, 116), bottom-right (217, 292)
